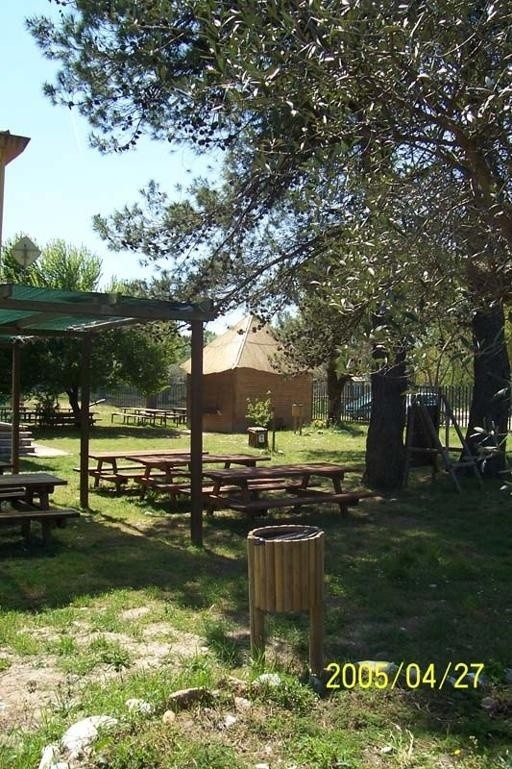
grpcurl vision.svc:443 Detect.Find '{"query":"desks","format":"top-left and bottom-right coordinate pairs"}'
top-left (0, 473), bottom-right (67, 540)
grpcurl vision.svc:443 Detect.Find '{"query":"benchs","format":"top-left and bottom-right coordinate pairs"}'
top-left (111, 406), bottom-right (187, 428)
top-left (1, 405), bottom-right (103, 433)
top-left (0, 491), bottom-right (38, 501)
top-left (73, 448), bottom-right (367, 526)
top-left (0, 509), bottom-right (79, 548)
